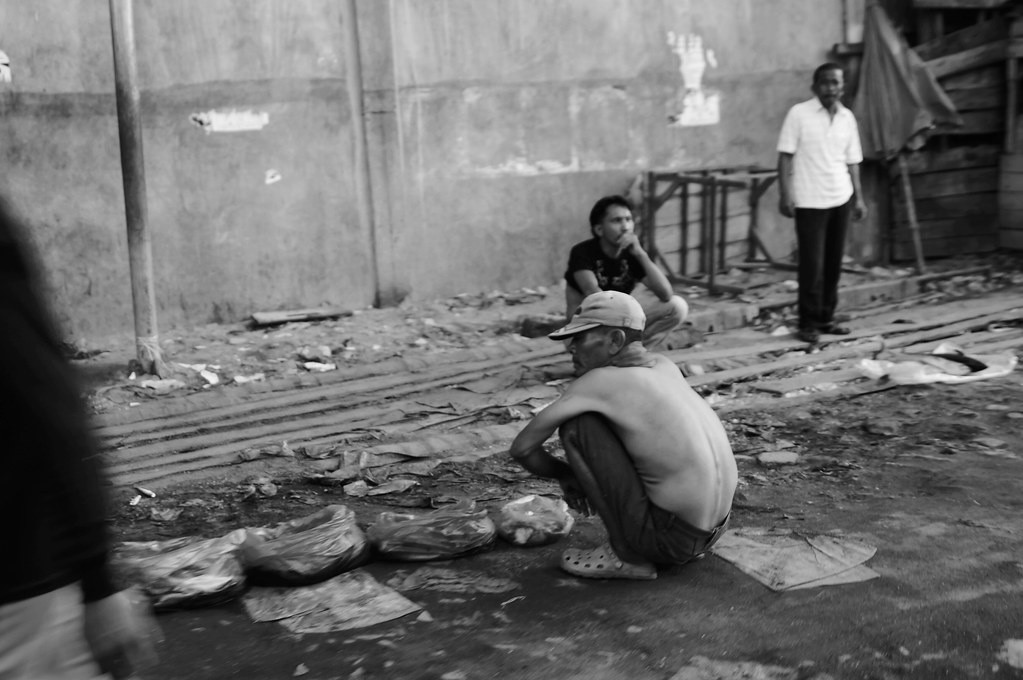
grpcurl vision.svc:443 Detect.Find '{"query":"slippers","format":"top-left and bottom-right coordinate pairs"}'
top-left (560, 541), bottom-right (658, 581)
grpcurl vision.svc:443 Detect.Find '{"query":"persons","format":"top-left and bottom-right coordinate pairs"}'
top-left (0, 198), bottom-right (160, 680)
top-left (561, 194), bottom-right (690, 351)
top-left (509, 290), bottom-right (739, 579)
top-left (774, 62), bottom-right (870, 345)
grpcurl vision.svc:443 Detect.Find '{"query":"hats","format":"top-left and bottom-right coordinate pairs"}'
top-left (546, 290), bottom-right (647, 341)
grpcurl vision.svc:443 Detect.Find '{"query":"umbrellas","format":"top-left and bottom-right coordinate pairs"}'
top-left (847, 0), bottom-right (965, 279)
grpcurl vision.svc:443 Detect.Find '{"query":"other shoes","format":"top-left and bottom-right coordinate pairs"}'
top-left (798, 326), bottom-right (821, 343)
top-left (818, 321), bottom-right (851, 335)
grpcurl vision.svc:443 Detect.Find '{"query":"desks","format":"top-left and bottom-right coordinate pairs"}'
top-left (639, 162), bottom-right (799, 297)
